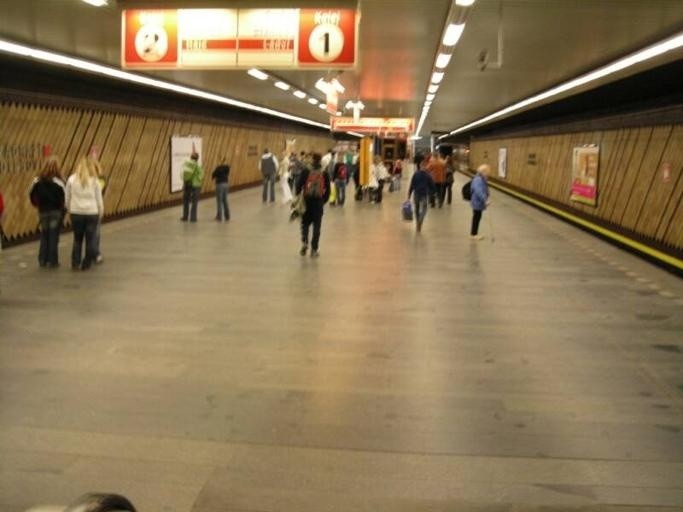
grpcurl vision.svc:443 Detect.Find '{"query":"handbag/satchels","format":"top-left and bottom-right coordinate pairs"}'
top-left (185, 179), bottom-right (192, 189)
top-left (462, 181), bottom-right (471, 200)
top-left (337, 165), bottom-right (348, 180)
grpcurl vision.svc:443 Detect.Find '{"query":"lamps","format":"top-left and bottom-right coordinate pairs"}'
top-left (415, 0), bottom-right (475, 135)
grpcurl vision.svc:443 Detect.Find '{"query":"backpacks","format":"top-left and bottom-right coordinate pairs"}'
top-left (304, 164), bottom-right (326, 199)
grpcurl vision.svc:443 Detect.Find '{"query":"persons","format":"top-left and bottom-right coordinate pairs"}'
top-left (92, 156), bottom-right (106, 264)
top-left (179, 152), bottom-right (203, 222)
top-left (577, 154), bottom-right (588, 179)
top-left (63, 155), bottom-right (103, 272)
top-left (256, 145), bottom-right (490, 258)
top-left (28, 154), bottom-right (65, 268)
top-left (211, 154), bottom-right (230, 222)
top-left (0, 189), bottom-right (3, 237)
